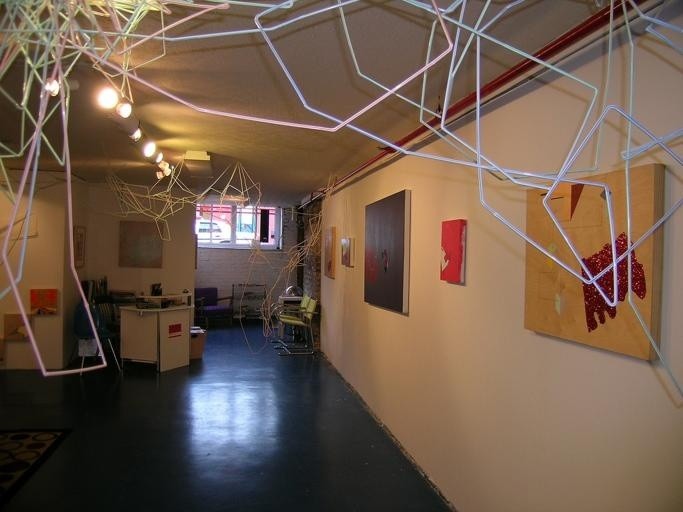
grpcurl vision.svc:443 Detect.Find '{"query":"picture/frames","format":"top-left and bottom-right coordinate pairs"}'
top-left (72, 225), bottom-right (86, 269)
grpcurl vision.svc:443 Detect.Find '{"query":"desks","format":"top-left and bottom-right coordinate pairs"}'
top-left (119, 305), bottom-right (194, 373)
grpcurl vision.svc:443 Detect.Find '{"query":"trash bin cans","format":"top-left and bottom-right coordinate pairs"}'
top-left (190, 327), bottom-right (205, 360)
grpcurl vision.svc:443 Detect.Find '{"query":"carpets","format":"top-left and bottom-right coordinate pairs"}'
top-left (0, 427), bottom-right (72, 512)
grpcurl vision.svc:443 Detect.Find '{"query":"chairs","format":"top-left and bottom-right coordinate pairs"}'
top-left (267, 293), bottom-right (319, 356)
top-left (73, 295), bottom-right (124, 377)
top-left (194, 286), bottom-right (233, 329)
